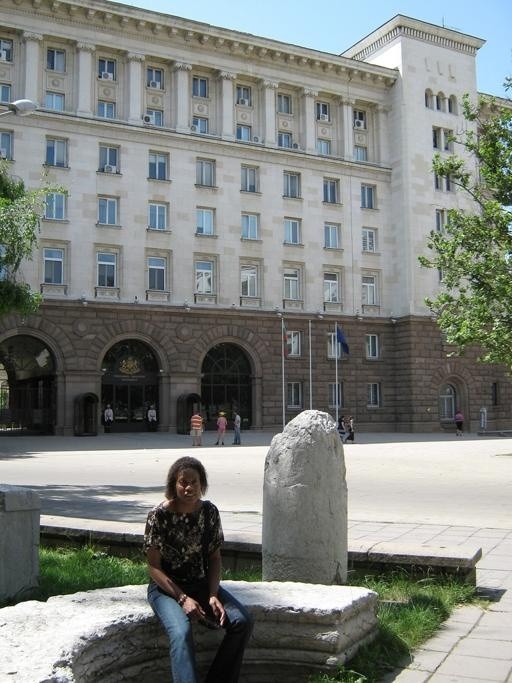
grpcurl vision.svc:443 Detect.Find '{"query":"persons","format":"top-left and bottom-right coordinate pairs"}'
top-left (190, 410), bottom-right (206, 446)
top-left (231, 409), bottom-right (242, 445)
top-left (214, 411), bottom-right (227, 445)
top-left (147, 403), bottom-right (157, 432)
top-left (103, 402), bottom-right (116, 432)
top-left (141, 456), bottom-right (253, 682)
top-left (345, 414), bottom-right (356, 444)
top-left (337, 415), bottom-right (347, 444)
top-left (454, 408), bottom-right (464, 436)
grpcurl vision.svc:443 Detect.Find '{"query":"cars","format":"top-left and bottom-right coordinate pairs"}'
top-left (100, 401), bottom-right (149, 421)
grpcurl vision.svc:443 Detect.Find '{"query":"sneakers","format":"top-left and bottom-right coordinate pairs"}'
top-left (232, 442), bottom-right (240, 445)
top-left (192, 443), bottom-right (202, 446)
top-left (216, 441), bottom-right (224, 445)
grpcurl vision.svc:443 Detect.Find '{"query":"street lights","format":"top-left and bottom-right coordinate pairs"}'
top-left (0, 95), bottom-right (39, 119)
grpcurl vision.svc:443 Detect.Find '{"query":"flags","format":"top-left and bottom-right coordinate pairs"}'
top-left (337, 325), bottom-right (349, 354)
top-left (282, 320), bottom-right (290, 360)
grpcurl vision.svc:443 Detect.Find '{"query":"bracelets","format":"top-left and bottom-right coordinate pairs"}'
top-left (177, 593), bottom-right (187, 603)
top-left (208, 591), bottom-right (217, 597)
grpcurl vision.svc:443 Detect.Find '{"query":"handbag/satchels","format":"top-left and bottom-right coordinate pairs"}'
top-left (158, 578), bottom-right (220, 630)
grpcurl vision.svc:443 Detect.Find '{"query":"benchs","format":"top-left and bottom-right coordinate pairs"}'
top-left (2, 580), bottom-right (382, 680)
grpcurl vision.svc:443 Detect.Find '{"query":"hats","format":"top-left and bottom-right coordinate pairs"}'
top-left (219, 412), bottom-right (225, 416)
